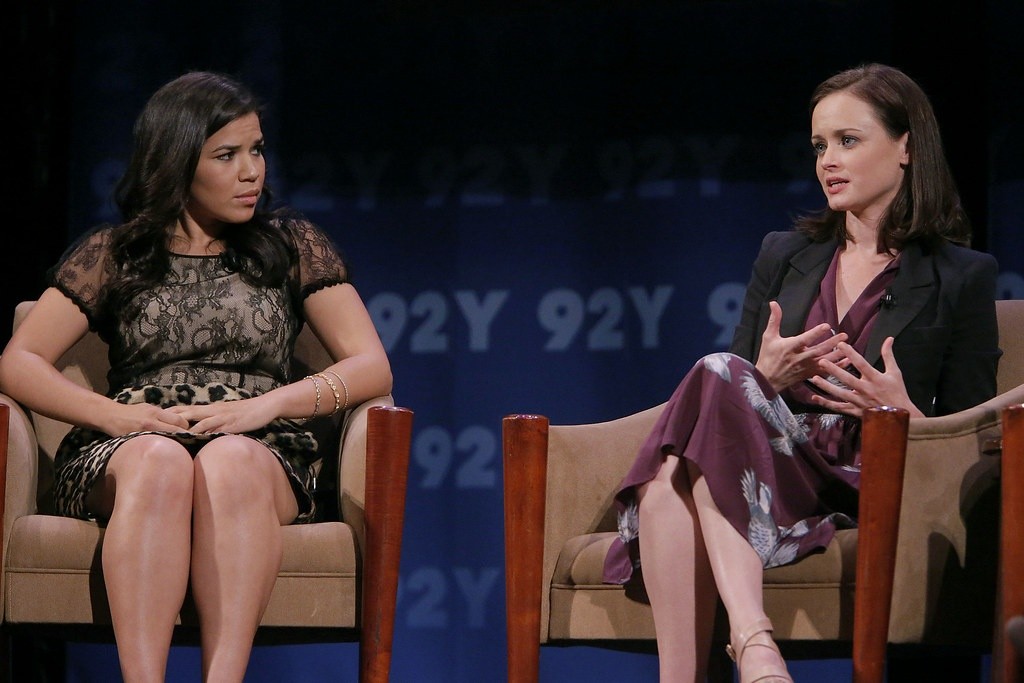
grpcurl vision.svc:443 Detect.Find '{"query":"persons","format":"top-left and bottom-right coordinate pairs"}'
top-left (600, 63), bottom-right (1007, 682)
top-left (1, 70), bottom-right (397, 683)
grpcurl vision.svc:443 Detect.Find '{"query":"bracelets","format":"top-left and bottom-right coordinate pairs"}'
top-left (301, 367), bottom-right (349, 423)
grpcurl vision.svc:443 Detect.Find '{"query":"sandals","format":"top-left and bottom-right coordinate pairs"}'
top-left (726, 615), bottom-right (796, 683)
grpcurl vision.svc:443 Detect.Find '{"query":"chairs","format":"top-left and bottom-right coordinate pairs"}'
top-left (501, 299), bottom-right (1024, 683)
top-left (0, 299), bottom-right (414, 683)
